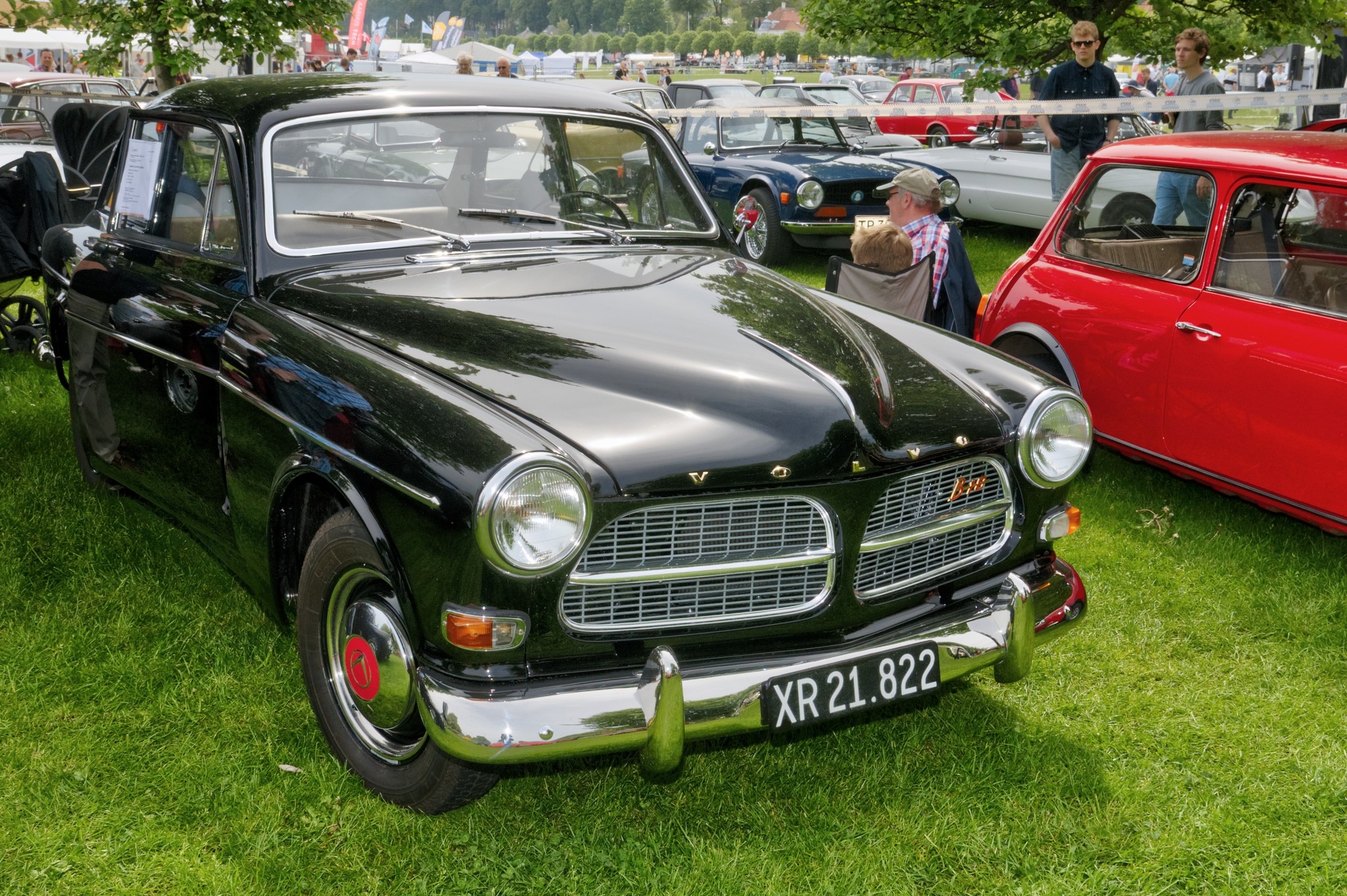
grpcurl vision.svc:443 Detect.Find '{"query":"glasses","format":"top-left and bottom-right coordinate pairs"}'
top-left (498, 66), bottom-right (506, 69)
top-left (1073, 40), bottom-right (1096, 48)
top-left (885, 190), bottom-right (898, 200)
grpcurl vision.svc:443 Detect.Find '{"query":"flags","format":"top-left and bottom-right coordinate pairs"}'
top-left (422, 20), bottom-right (433, 34)
top-left (361, 31), bottom-right (371, 44)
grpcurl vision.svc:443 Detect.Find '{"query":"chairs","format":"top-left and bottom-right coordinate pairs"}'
top-left (998, 130), bottom-right (1028, 151)
top-left (825, 251), bottom-right (936, 324)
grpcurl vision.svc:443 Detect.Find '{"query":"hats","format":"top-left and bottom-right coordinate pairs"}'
top-left (637, 61), bottom-right (645, 67)
top-left (1120, 80), bottom-right (1146, 90)
top-left (876, 167), bottom-right (941, 198)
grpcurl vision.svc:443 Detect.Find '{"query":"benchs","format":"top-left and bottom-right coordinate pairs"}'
top-left (1067, 228), bottom-right (1312, 307)
top-left (168, 206), bottom-right (453, 254)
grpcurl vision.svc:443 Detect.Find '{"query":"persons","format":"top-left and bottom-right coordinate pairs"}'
top-left (56, 56), bottom-right (66, 73)
top-left (130, 59), bottom-right (147, 78)
top-left (310, 61), bottom-right (319, 73)
top-left (1151, 27), bottom-right (1226, 228)
top-left (1125, 57), bottom-right (1289, 125)
top-left (305, 59), bottom-right (313, 73)
top-left (6, 53), bottom-right (17, 63)
top-left (850, 221), bottom-right (915, 277)
top-left (345, 48), bottom-right (357, 72)
top-left (284, 62), bottom-right (293, 73)
top-left (73, 63), bottom-right (84, 75)
top-left (314, 59), bottom-right (327, 72)
top-left (64, 57), bottom-right (74, 73)
top-left (613, 56), bottom-right (1050, 101)
top-left (334, 58), bottom-right (351, 72)
top-left (494, 57), bottom-right (519, 80)
top-left (876, 167), bottom-right (951, 310)
top-left (1036, 20), bottom-right (1123, 203)
top-left (297, 60), bottom-right (302, 73)
top-left (17, 51), bottom-right (28, 66)
top-left (29, 48), bottom-right (60, 74)
top-left (451, 53), bottom-right (475, 77)
top-left (134, 53), bottom-right (142, 63)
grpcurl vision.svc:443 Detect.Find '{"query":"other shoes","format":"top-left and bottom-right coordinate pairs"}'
top-left (1273, 115), bottom-right (1280, 120)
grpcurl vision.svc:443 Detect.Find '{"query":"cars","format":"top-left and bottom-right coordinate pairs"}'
top-left (879, 114), bottom-right (1321, 240)
top-left (1112, 71), bottom-right (1133, 91)
top-left (975, 124), bottom-right (1347, 545)
top-left (624, 94), bottom-right (967, 268)
top-left (1283, 119), bottom-right (1347, 235)
top-left (875, 80), bottom-right (1039, 149)
top-left (29, 73), bottom-right (1094, 816)
top-left (1, 74), bottom-right (974, 278)
top-left (494, 77), bottom-right (731, 199)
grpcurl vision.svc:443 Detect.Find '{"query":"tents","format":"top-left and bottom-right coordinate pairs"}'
top-left (1106, 33), bottom-right (1323, 90)
top-left (0, 26), bottom-right (675, 83)
